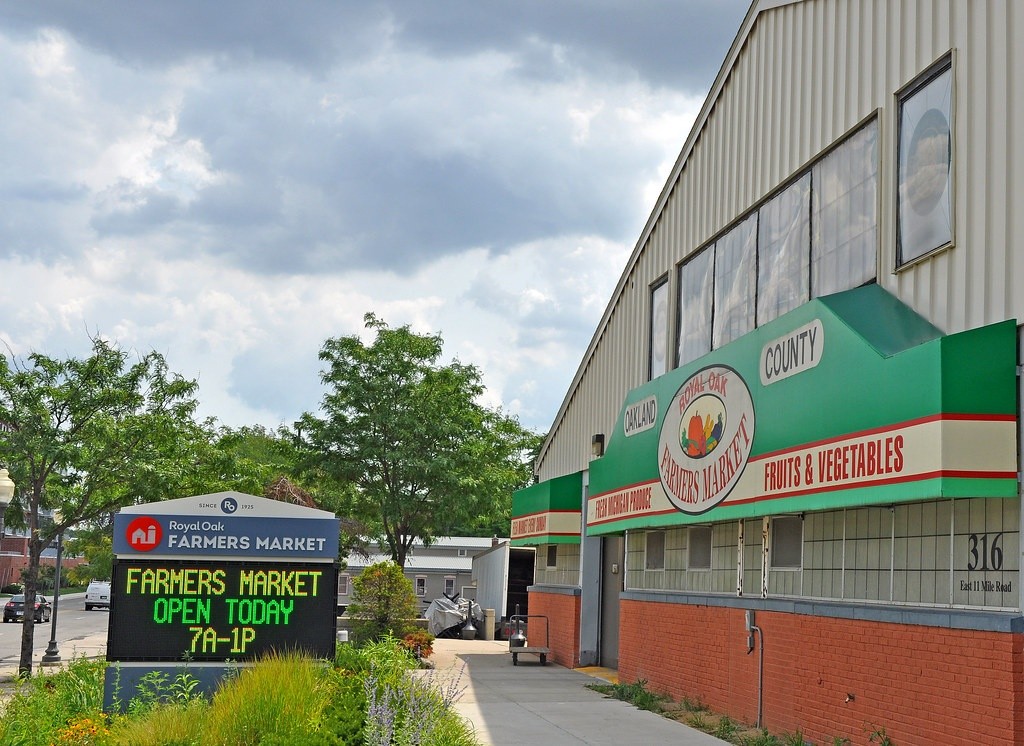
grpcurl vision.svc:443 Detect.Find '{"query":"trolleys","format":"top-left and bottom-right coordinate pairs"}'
top-left (507, 614), bottom-right (550, 665)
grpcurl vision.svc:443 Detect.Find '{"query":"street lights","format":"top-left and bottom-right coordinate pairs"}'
top-left (40, 506), bottom-right (68, 667)
top-left (0, 469), bottom-right (16, 542)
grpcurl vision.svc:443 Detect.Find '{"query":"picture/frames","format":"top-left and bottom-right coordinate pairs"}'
top-left (892, 47), bottom-right (956, 275)
top-left (673, 107), bottom-right (882, 370)
top-left (647, 269), bottom-right (671, 383)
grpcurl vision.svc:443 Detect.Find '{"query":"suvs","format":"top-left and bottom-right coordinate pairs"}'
top-left (84, 579), bottom-right (111, 611)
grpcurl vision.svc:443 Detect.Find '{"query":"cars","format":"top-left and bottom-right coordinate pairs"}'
top-left (3, 595), bottom-right (52, 623)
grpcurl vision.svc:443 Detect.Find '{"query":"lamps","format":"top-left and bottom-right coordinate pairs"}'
top-left (591, 433), bottom-right (604, 457)
